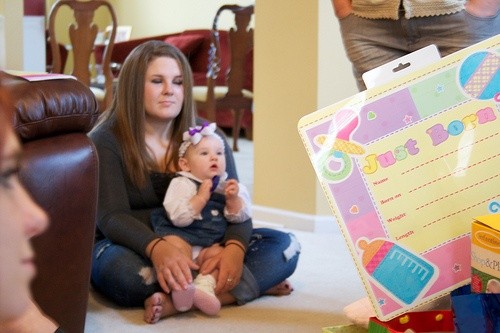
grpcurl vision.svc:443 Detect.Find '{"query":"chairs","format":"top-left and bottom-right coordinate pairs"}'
top-left (192, 4), bottom-right (254, 151)
top-left (49, 0), bottom-right (117, 114)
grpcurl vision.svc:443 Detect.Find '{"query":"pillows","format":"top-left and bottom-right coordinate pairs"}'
top-left (164, 35), bottom-right (204, 61)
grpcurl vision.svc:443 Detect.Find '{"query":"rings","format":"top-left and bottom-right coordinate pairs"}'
top-left (226, 279), bottom-right (234, 283)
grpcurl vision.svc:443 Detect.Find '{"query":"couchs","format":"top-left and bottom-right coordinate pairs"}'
top-left (15, 76), bottom-right (100, 333)
top-left (46, 29), bottom-right (253, 127)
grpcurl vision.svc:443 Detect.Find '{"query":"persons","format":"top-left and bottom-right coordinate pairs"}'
top-left (0, 83), bottom-right (68, 333)
top-left (330, 0), bottom-right (500, 95)
top-left (87, 40), bottom-right (300, 324)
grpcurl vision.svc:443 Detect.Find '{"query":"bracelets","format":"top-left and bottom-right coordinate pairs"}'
top-left (224, 242), bottom-right (246, 253)
top-left (148, 237), bottom-right (167, 258)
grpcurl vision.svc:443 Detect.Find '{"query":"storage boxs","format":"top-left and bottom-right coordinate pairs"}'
top-left (471, 214), bottom-right (500, 293)
